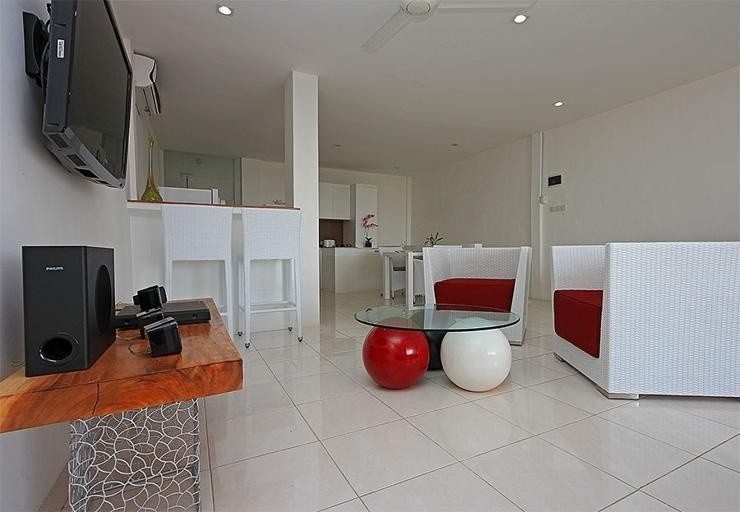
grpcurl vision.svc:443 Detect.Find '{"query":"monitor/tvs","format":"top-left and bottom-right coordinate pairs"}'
top-left (22, 1), bottom-right (133, 190)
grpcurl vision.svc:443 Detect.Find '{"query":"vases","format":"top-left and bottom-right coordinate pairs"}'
top-left (141, 130), bottom-right (164, 202)
top-left (365, 236), bottom-right (373, 247)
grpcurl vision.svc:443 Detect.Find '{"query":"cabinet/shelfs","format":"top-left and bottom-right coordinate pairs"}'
top-left (319, 182), bottom-right (352, 222)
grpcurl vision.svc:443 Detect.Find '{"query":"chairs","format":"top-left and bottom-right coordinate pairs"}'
top-left (379, 244), bottom-right (463, 305)
top-left (549, 241), bottom-right (740, 401)
top-left (422, 246), bottom-right (533, 346)
top-left (160, 205), bottom-right (302, 348)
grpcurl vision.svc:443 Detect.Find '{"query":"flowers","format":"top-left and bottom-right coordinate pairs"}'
top-left (361, 214), bottom-right (379, 238)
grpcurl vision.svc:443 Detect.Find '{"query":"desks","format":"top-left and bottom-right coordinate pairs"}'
top-left (0, 296), bottom-right (243, 510)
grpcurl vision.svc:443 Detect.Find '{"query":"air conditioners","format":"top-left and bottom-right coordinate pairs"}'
top-left (132, 52), bottom-right (166, 122)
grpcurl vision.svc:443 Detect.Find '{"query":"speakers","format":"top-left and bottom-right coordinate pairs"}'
top-left (143, 316), bottom-right (183, 356)
top-left (135, 307), bottom-right (165, 339)
top-left (137, 285), bottom-right (164, 310)
top-left (21, 245), bottom-right (116, 378)
top-left (133, 285), bottom-right (167, 305)
